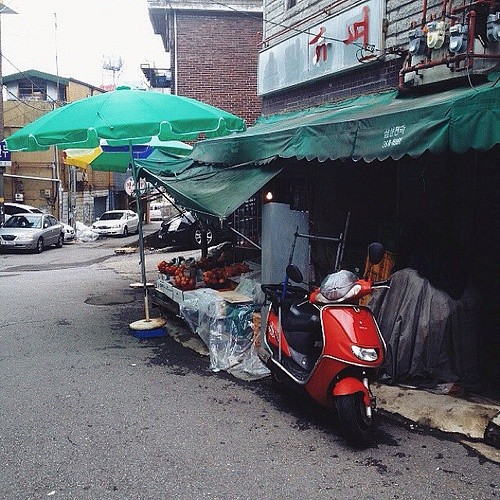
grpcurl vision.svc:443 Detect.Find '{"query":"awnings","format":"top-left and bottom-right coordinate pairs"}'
top-left (187, 72), bottom-right (500, 167)
top-left (132, 148), bottom-right (287, 228)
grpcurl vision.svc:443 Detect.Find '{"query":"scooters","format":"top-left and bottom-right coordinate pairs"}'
top-left (255, 242), bottom-right (395, 448)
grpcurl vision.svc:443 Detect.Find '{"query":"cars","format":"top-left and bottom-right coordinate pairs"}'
top-left (156, 196), bottom-right (257, 248)
top-left (91, 209), bottom-right (143, 238)
top-left (4, 200), bottom-right (76, 243)
top-left (0, 211), bottom-right (66, 254)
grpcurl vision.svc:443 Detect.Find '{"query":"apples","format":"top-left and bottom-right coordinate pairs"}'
top-left (158, 259), bottom-right (248, 285)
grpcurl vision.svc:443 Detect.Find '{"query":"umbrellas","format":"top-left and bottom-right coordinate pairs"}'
top-left (62, 135), bottom-right (194, 283)
top-left (2, 89), bottom-right (247, 322)
